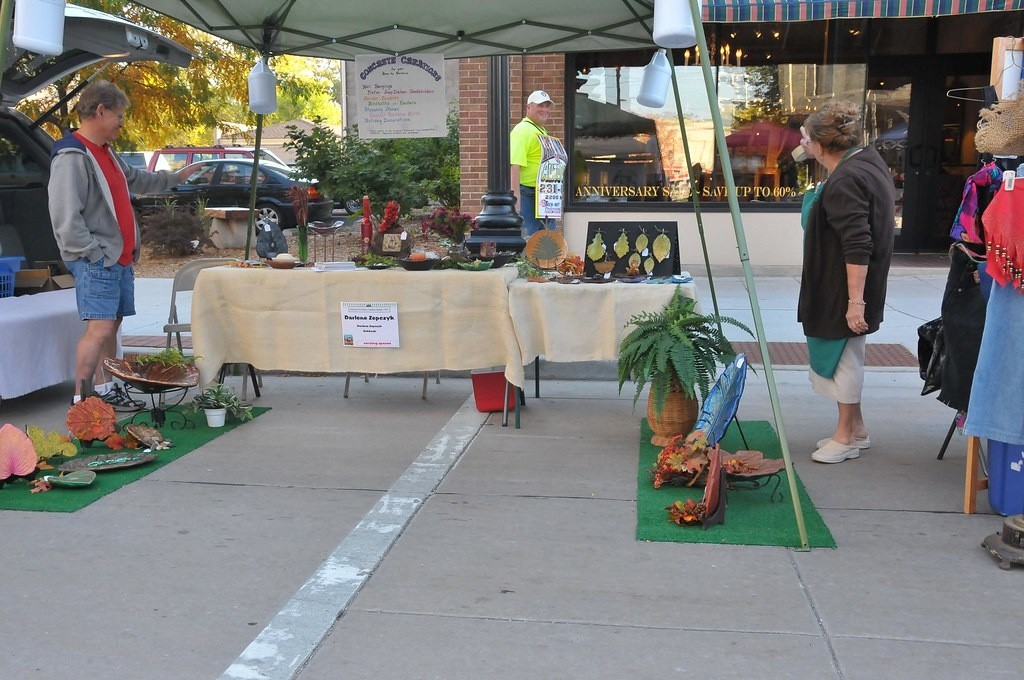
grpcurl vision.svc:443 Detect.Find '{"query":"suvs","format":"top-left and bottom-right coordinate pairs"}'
top-left (115, 150), bottom-right (170, 172)
top-left (0, 0), bottom-right (203, 291)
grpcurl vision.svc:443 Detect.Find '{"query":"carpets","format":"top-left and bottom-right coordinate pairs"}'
top-left (0, 402), bottom-right (273, 513)
top-left (635, 418), bottom-right (838, 548)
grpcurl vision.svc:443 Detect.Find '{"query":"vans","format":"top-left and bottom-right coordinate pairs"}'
top-left (148, 148), bottom-right (290, 182)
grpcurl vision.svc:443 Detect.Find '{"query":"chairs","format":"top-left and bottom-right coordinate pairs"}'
top-left (161, 258), bottom-right (263, 402)
top-left (947, 35), bottom-right (1024, 103)
top-left (702, 119), bottom-right (788, 204)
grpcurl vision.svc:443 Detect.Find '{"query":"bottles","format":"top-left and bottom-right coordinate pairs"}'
top-left (637, 49), bottom-right (673, 107)
top-left (653, 0), bottom-right (701, 47)
top-left (248, 56), bottom-right (277, 114)
top-left (12, 0), bottom-right (65, 55)
top-left (361, 196), bottom-right (372, 262)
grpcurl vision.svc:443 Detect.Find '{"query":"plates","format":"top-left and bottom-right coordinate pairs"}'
top-left (59, 452), bottom-right (155, 470)
top-left (366, 266), bottom-right (389, 269)
top-left (47, 471), bottom-right (95, 485)
top-left (526, 230), bottom-right (568, 268)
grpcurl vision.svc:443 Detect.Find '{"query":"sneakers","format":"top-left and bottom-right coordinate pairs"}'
top-left (811, 440), bottom-right (859, 463)
top-left (817, 433), bottom-right (870, 450)
top-left (70, 392), bottom-right (118, 412)
top-left (100, 383), bottom-right (147, 411)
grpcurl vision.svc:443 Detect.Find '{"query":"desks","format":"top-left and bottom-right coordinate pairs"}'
top-left (0, 287), bottom-right (123, 401)
top-left (191, 264), bottom-right (695, 430)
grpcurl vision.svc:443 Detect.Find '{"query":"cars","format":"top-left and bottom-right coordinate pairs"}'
top-left (130, 158), bottom-right (330, 234)
top-left (288, 163), bottom-right (363, 215)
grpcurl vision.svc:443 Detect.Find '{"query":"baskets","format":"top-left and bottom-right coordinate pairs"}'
top-left (0, 256), bottom-right (26, 298)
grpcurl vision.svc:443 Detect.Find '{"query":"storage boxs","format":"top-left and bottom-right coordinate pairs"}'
top-left (0, 254), bottom-right (76, 297)
top-left (469, 367), bottom-right (516, 414)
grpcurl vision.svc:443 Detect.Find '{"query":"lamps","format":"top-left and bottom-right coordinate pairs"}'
top-left (685, 23), bottom-right (743, 67)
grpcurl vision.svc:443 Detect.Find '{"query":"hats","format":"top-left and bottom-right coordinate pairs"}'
top-left (973, 80), bottom-right (1024, 156)
top-left (528, 90), bottom-right (554, 106)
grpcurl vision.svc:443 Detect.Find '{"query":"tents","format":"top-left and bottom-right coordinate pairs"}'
top-left (123, 0), bottom-right (811, 553)
top-left (724, 121), bottom-right (803, 165)
top-left (574, 92), bottom-right (671, 202)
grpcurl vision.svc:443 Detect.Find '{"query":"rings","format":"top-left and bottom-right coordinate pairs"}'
top-left (855, 322), bottom-right (860, 325)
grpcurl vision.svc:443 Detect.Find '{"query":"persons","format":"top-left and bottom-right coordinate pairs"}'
top-left (48, 80), bottom-right (146, 412)
top-left (509, 90), bottom-right (556, 237)
top-left (797, 98), bottom-right (896, 464)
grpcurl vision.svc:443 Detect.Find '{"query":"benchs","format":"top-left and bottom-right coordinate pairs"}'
top-left (205, 208), bottom-right (260, 249)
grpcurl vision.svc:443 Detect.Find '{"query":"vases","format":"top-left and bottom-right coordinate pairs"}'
top-left (447, 241), bottom-right (469, 257)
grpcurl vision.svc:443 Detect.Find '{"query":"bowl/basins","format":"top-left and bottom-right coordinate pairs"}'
top-left (469, 251), bottom-right (516, 268)
top-left (264, 259), bottom-right (301, 268)
top-left (401, 258), bottom-right (441, 271)
top-left (457, 260), bottom-right (494, 271)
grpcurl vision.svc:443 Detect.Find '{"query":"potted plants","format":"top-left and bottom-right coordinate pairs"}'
top-left (618, 285), bottom-right (758, 447)
top-left (183, 377), bottom-right (255, 428)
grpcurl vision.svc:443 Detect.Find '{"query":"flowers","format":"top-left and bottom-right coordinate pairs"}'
top-left (378, 202), bottom-right (401, 233)
top-left (663, 497), bottom-right (706, 526)
top-left (646, 429), bottom-right (711, 489)
top-left (421, 206), bottom-right (479, 247)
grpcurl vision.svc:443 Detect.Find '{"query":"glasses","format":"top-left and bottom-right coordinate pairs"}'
top-left (106, 106), bottom-right (125, 122)
top-left (805, 139), bottom-right (820, 146)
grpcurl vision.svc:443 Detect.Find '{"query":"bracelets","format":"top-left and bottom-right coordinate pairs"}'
top-left (848, 300), bottom-right (867, 305)
